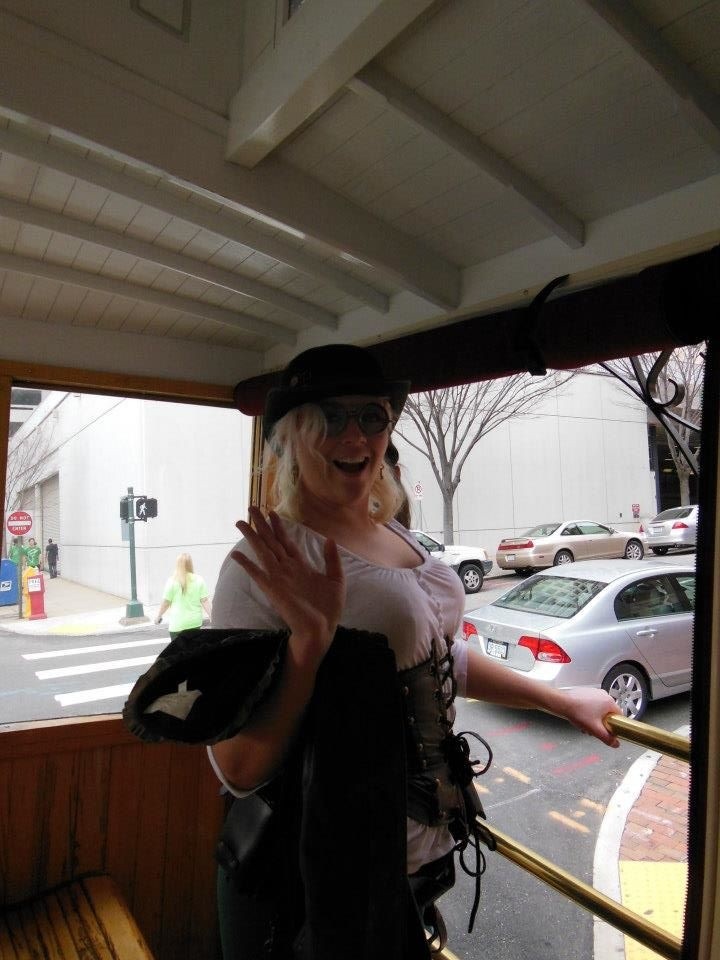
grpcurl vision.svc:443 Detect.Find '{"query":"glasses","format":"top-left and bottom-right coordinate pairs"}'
top-left (299, 402), bottom-right (397, 437)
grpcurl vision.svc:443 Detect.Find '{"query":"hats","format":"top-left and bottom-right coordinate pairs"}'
top-left (263, 343), bottom-right (412, 458)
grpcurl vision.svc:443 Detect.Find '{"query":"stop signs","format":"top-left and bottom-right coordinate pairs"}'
top-left (7, 511), bottom-right (33, 537)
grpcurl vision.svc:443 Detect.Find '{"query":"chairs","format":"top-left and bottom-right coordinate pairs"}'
top-left (633, 585), bottom-right (660, 616)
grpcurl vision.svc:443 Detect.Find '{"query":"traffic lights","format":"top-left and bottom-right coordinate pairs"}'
top-left (136, 498), bottom-right (157, 518)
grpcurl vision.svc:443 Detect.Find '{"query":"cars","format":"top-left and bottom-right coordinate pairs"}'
top-left (646, 504), bottom-right (699, 555)
top-left (496, 520), bottom-right (648, 572)
top-left (455, 559), bottom-right (696, 722)
top-left (409, 528), bottom-right (493, 594)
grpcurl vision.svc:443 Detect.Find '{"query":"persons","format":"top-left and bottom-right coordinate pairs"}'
top-left (207, 343), bottom-right (625, 960)
top-left (8, 538), bottom-right (26, 570)
top-left (25, 538), bottom-right (41, 570)
top-left (154, 552), bottom-right (212, 642)
top-left (45, 539), bottom-right (59, 579)
